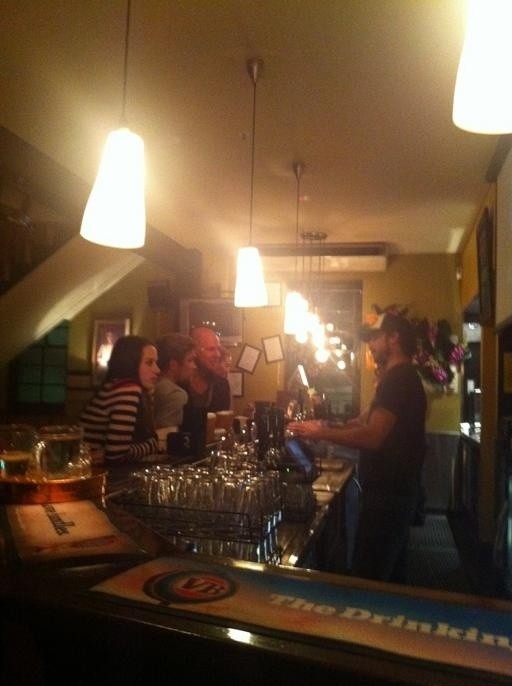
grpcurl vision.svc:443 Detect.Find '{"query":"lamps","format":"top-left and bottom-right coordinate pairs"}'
top-left (79, 3), bottom-right (146, 250)
top-left (234, 63), bottom-right (267, 308)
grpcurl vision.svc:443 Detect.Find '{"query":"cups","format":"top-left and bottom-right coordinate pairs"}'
top-left (167, 530), bottom-right (277, 561)
top-left (122, 449), bottom-right (280, 522)
top-left (0, 423), bottom-right (93, 483)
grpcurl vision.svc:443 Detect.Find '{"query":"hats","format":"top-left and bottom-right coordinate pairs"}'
top-left (358, 312), bottom-right (411, 343)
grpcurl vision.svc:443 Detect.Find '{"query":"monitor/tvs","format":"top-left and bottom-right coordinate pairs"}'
top-left (285, 435), bottom-right (318, 484)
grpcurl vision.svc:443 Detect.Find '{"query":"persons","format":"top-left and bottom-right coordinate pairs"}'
top-left (76, 336), bottom-right (162, 464)
top-left (183, 324), bottom-right (231, 459)
top-left (283, 308), bottom-right (425, 584)
top-left (212, 346), bottom-right (230, 376)
top-left (148, 328), bottom-right (202, 450)
